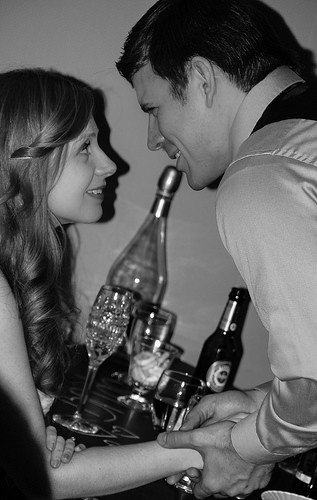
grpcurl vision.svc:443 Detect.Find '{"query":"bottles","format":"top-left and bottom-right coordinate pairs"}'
top-left (105, 166), bottom-right (183, 308)
top-left (194, 287), bottom-right (252, 399)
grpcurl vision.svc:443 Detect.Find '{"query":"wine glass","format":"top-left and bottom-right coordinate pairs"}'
top-left (52, 285), bottom-right (133, 434)
top-left (118, 334), bottom-right (180, 410)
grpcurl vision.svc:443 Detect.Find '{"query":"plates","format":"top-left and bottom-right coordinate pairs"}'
top-left (261, 490), bottom-right (317, 500)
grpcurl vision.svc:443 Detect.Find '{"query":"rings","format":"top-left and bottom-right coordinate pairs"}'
top-left (236, 496), bottom-right (245, 499)
top-left (71, 437), bottom-right (75, 441)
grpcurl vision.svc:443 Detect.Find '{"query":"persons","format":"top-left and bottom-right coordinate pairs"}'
top-left (115, 0), bottom-right (317, 500)
top-left (0, 69), bottom-right (252, 500)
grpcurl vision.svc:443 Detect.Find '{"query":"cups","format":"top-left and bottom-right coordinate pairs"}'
top-left (126, 305), bottom-right (176, 385)
top-left (152, 368), bottom-right (195, 442)
